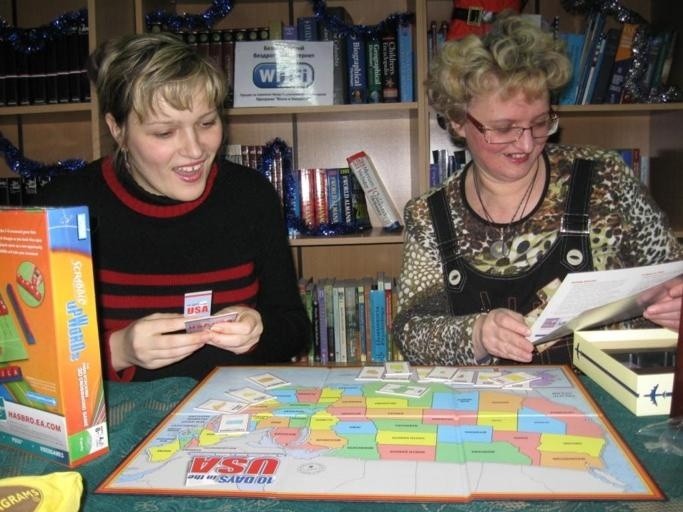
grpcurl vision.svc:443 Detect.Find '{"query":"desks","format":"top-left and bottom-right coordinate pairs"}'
top-left (0, 371), bottom-right (682, 510)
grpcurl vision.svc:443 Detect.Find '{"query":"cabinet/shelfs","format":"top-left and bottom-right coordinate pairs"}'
top-left (0, 2), bottom-right (682, 279)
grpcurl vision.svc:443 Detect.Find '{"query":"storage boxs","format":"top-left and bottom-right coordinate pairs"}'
top-left (227, 38), bottom-right (345, 108)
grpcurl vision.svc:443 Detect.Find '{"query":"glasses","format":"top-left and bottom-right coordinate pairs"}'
top-left (465, 107), bottom-right (561, 145)
